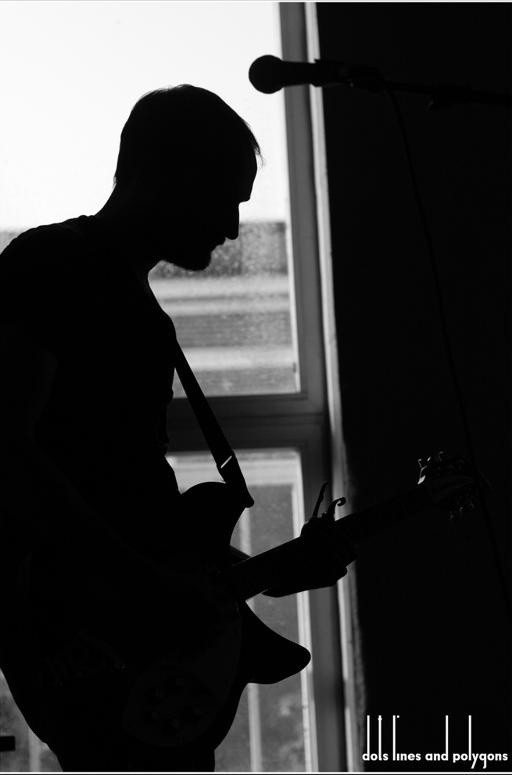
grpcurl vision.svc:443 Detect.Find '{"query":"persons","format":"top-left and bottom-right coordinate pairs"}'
top-left (0, 85), bottom-right (354, 771)
